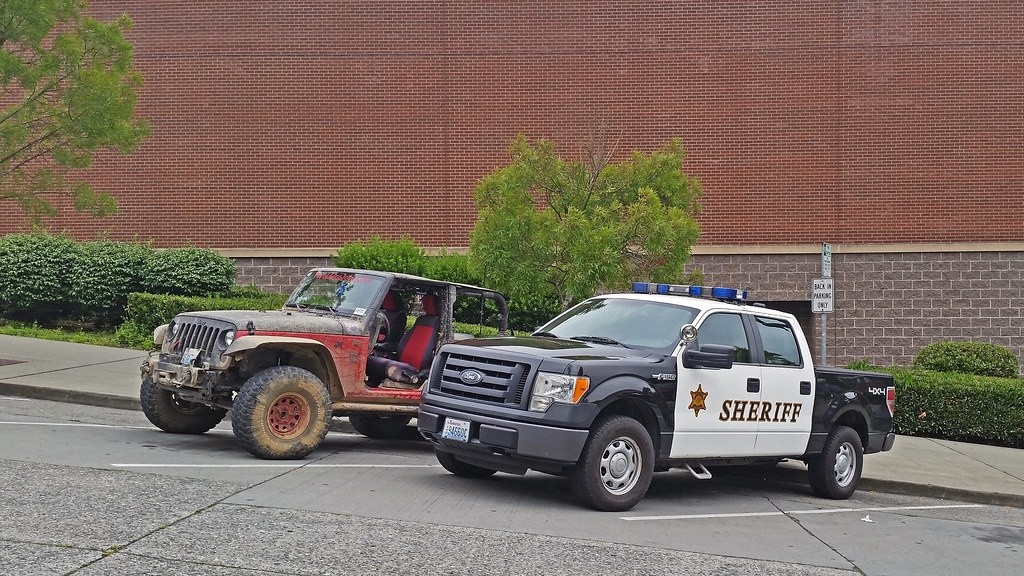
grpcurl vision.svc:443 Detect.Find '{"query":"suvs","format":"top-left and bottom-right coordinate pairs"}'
top-left (139, 266), bottom-right (512, 460)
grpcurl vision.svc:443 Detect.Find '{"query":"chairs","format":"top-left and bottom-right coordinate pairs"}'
top-left (371, 291), bottom-right (406, 359)
top-left (366, 292), bottom-right (441, 385)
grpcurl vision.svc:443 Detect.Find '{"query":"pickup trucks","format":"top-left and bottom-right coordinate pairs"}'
top-left (415, 279), bottom-right (899, 515)
